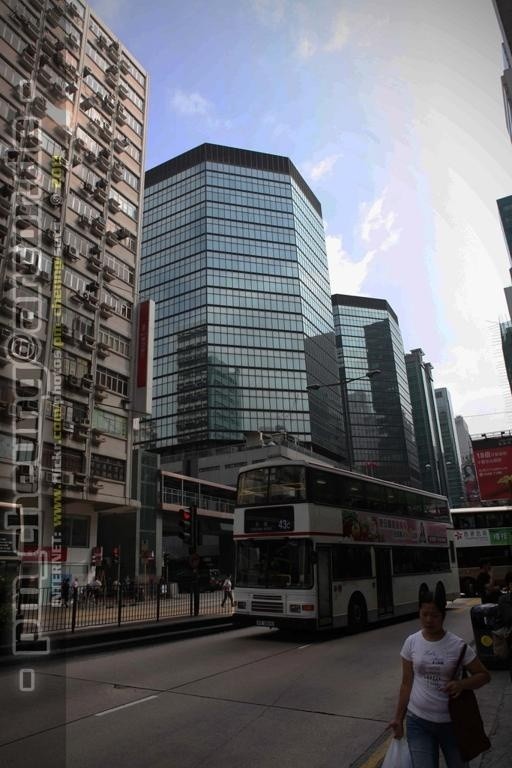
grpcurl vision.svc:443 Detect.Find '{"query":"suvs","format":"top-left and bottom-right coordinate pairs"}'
top-left (175, 569), bottom-right (222, 593)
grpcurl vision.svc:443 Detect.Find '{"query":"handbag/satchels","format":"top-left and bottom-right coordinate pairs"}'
top-left (492, 627), bottom-right (512, 659)
top-left (449, 673), bottom-right (491, 762)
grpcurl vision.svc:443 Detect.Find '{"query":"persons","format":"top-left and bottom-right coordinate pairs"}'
top-left (384, 591), bottom-right (492, 768)
top-left (477, 565), bottom-right (494, 593)
top-left (72, 575), bottom-right (131, 606)
top-left (220, 573), bottom-right (235, 607)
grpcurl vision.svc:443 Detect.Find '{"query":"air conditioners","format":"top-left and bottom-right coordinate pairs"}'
top-left (0, 0), bottom-right (131, 493)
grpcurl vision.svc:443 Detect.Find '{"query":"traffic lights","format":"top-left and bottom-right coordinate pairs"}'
top-left (113, 545), bottom-right (120, 565)
top-left (178, 508), bottom-right (194, 546)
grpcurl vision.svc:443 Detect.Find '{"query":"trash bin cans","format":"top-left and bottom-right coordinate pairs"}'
top-left (470, 602), bottom-right (509, 671)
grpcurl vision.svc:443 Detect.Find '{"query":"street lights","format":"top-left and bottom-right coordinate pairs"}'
top-left (425, 460), bottom-right (453, 495)
top-left (306, 366), bottom-right (382, 473)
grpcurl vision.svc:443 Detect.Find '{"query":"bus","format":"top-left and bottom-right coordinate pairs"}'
top-left (231, 459), bottom-right (461, 633)
top-left (449, 506), bottom-right (511, 598)
